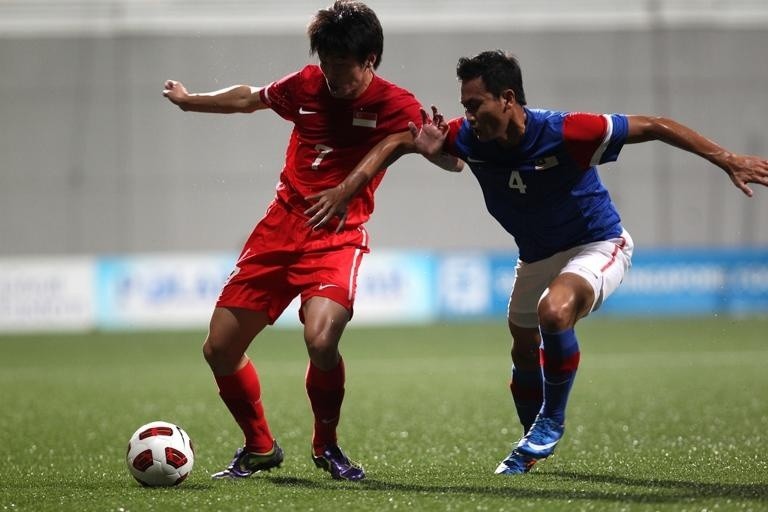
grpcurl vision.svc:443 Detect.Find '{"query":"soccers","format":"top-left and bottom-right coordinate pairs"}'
top-left (126, 422), bottom-right (196, 485)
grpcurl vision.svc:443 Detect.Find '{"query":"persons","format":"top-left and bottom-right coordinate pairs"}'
top-left (302, 50), bottom-right (767, 477)
top-left (163, 1), bottom-right (466, 483)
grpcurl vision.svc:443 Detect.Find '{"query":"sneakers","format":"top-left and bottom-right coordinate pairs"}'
top-left (494, 448), bottom-right (540, 475)
top-left (518, 411), bottom-right (564, 459)
top-left (211, 439), bottom-right (284, 480)
top-left (311, 446), bottom-right (365, 482)
